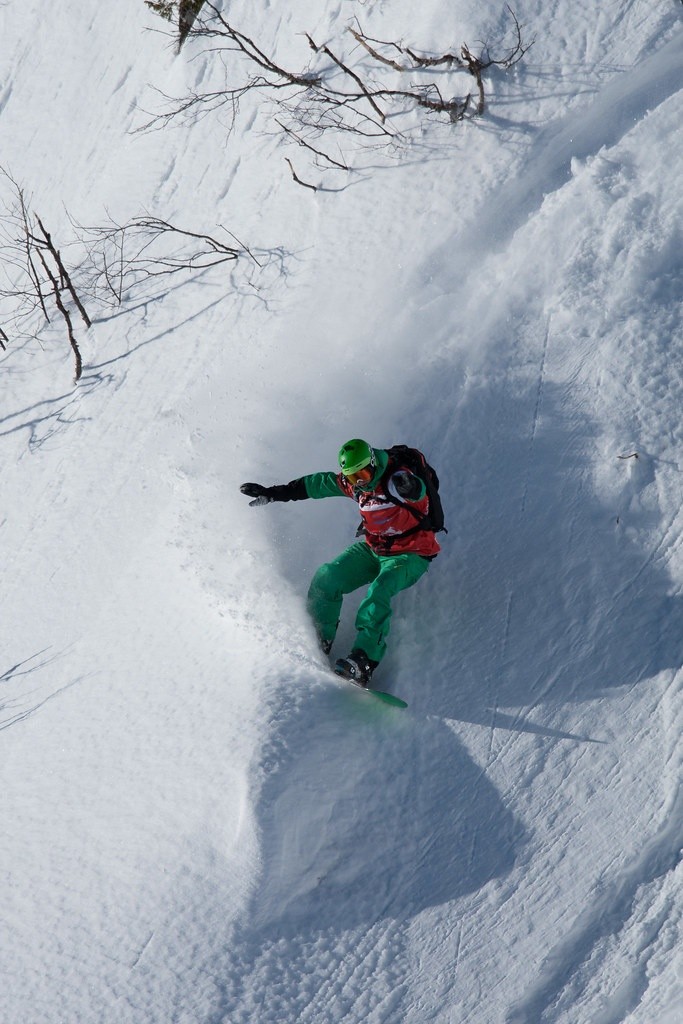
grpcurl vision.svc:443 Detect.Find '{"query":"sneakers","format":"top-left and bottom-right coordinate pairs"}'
top-left (317, 638), bottom-right (335, 656)
top-left (334, 650), bottom-right (379, 688)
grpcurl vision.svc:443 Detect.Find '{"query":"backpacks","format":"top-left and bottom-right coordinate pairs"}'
top-left (384, 446), bottom-right (449, 537)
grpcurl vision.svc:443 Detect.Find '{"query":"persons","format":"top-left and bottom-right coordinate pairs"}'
top-left (239, 439), bottom-right (441, 688)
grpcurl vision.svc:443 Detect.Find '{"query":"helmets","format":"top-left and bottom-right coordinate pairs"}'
top-left (337, 439), bottom-right (377, 477)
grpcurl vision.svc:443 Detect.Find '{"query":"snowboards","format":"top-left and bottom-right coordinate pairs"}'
top-left (218, 608), bottom-right (410, 710)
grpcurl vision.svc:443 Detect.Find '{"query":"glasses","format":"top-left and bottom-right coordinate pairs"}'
top-left (346, 464), bottom-right (376, 486)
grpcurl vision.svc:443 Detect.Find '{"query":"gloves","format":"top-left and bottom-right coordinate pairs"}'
top-left (391, 471), bottom-right (412, 494)
top-left (239, 482), bottom-right (288, 507)
